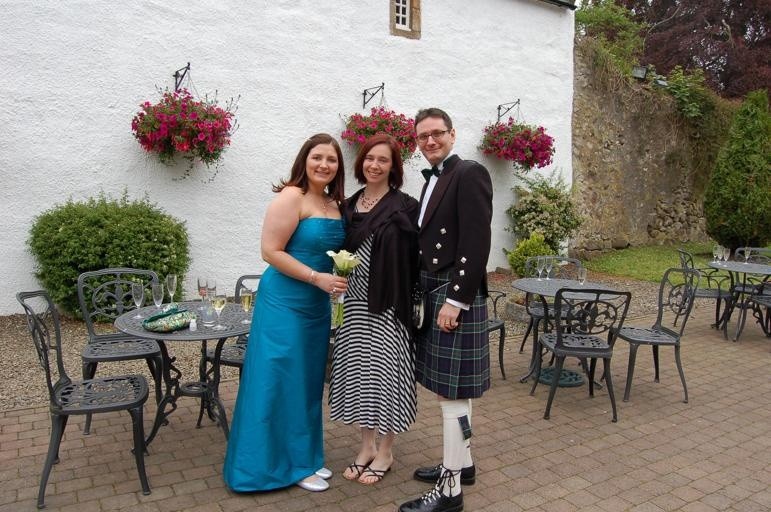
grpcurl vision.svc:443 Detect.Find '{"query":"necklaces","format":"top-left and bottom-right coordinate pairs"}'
top-left (358, 185), bottom-right (392, 209)
top-left (306, 191), bottom-right (331, 210)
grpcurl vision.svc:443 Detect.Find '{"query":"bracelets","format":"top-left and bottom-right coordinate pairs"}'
top-left (309, 268), bottom-right (317, 284)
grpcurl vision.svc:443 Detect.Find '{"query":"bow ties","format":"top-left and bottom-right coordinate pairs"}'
top-left (421, 164), bottom-right (440, 181)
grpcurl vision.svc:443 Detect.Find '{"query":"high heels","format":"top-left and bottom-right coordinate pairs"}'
top-left (357, 455), bottom-right (393, 484)
top-left (342, 453), bottom-right (374, 480)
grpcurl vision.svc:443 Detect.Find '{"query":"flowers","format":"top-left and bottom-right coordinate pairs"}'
top-left (339, 106), bottom-right (418, 164)
top-left (131, 80), bottom-right (242, 185)
top-left (324, 249), bottom-right (361, 329)
top-left (479, 115), bottom-right (556, 174)
top-left (503, 166), bottom-right (585, 246)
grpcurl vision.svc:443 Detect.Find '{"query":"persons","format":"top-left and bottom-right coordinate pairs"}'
top-left (398, 106), bottom-right (494, 511)
top-left (221, 133), bottom-right (350, 495)
top-left (329, 130), bottom-right (418, 486)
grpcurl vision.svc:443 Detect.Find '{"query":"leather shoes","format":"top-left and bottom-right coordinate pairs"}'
top-left (297, 474), bottom-right (329, 492)
top-left (315, 467), bottom-right (332, 479)
top-left (398, 488), bottom-right (463, 512)
top-left (413, 463), bottom-right (475, 486)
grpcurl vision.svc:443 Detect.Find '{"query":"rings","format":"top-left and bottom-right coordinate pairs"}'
top-left (446, 323), bottom-right (450, 325)
top-left (333, 288), bottom-right (337, 292)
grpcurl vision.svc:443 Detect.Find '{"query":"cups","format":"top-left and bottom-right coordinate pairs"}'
top-left (152, 282), bottom-right (164, 308)
top-left (167, 274), bottom-right (177, 306)
top-left (132, 284), bottom-right (144, 319)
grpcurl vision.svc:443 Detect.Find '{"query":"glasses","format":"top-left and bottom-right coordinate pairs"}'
top-left (415, 130), bottom-right (450, 143)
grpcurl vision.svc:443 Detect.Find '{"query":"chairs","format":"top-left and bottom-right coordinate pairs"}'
top-left (483, 256), bottom-right (698, 425)
top-left (673, 248), bottom-right (770, 342)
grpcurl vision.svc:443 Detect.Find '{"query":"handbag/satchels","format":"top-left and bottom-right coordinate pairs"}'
top-left (411, 282), bottom-right (433, 339)
top-left (141, 302), bottom-right (197, 333)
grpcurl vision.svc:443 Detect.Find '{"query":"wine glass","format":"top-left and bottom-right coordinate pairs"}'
top-left (536, 257), bottom-right (587, 286)
top-left (197, 276), bottom-right (230, 332)
top-left (240, 287), bottom-right (252, 325)
top-left (712, 246), bottom-right (753, 268)
